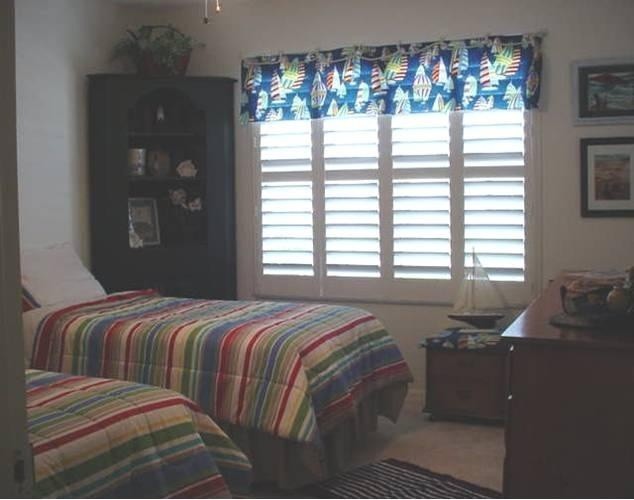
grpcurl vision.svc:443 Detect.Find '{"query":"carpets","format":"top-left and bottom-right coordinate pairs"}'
top-left (274, 456), bottom-right (506, 498)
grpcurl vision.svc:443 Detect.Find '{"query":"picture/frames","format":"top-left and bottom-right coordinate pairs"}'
top-left (580, 137), bottom-right (634, 217)
top-left (569, 56), bottom-right (634, 127)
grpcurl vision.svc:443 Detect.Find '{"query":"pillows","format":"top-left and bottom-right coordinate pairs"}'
top-left (20, 242), bottom-right (108, 308)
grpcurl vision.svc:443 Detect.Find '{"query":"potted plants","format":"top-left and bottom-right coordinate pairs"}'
top-left (105, 20), bottom-right (207, 77)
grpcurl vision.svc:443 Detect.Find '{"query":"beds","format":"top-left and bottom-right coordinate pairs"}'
top-left (22, 369), bottom-right (267, 498)
top-left (22, 289), bottom-right (413, 498)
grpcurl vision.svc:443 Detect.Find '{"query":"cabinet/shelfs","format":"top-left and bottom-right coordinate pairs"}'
top-left (501, 273), bottom-right (633, 500)
top-left (418, 328), bottom-right (507, 426)
top-left (85, 74), bottom-right (238, 300)
